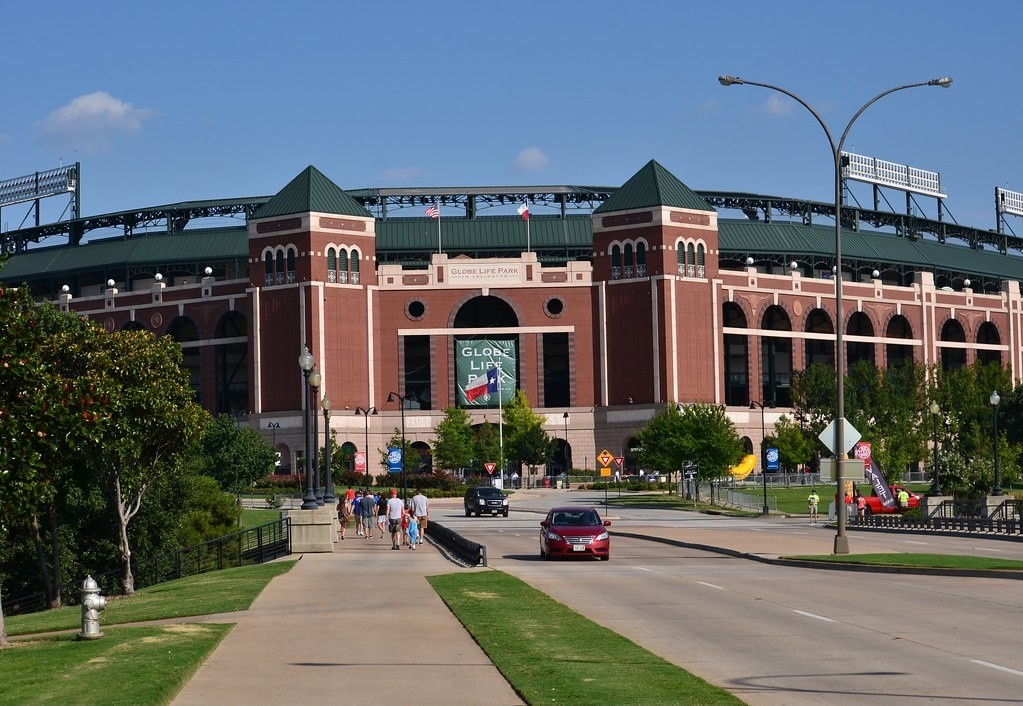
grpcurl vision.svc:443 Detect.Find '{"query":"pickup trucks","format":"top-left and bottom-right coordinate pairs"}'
top-left (860, 484), bottom-right (925, 518)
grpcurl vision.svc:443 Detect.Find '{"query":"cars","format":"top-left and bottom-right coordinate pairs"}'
top-left (539, 506), bottom-right (612, 562)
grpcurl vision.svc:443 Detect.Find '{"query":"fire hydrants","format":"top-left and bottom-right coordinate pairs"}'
top-left (76, 575), bottom-right (109, 640)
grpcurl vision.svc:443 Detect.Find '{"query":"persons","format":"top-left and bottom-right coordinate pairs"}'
top-left (898, 487), bottom-right (909, 506)
top-left (845, 493), bottom-right (852, 504)
top-left (511, 470), bottom-right (518, 489)
top-left (640, 468), bottom-right (644, 476)
top-left (855, 489), bottom-right (866, 515)
top-left (614, 469), bottom-right (620, 482)
top-left (503, 472), bottom-right (509, 489)
top-left (338, 485), bottom-right (430, 550)
top-left (808, 489), bottom-right (819, 524)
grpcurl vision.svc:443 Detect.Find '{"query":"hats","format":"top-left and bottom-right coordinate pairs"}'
top-left (390, 490), bottom-right (397, 494)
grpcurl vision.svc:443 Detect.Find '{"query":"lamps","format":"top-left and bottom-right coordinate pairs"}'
top-left (790, 261), bottom-right (798, 271)
top-left (746, 256), bottom-right (755, 267)
top-left (964, 279), bottom-right (971, 288)
top-left (62, 285), bottom-right (70, 294)
top-left (107, 279), bottom-right (116, 289)
top-left (661, 400), bottom-right (665, 404)
top-left (595, 403), bottom-right (599, 408)
top-left (628, 397), bottom-right (634, 403)
top-left (204, 267), bottom-right (213, 277)
top-left (872, 270), bottom-right (880, 279)
top-left (155, 273), bottom-right (163, 283)
top-left (832, 266), bottom-right (836, 275)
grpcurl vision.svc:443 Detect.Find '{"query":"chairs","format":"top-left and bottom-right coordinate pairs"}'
top-left (579, 515), bottom-right (587, 524)
top-left (554, 513), bottom-right (566, 524)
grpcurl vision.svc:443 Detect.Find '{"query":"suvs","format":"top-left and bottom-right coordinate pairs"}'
top-left (464, 486), bottom-right (509, 518)
top-left (640, 475), bottom-right (656, 483)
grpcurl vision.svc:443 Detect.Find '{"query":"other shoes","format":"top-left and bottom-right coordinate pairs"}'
top-left (412, 545), bottom-right (415, 550)
top-left (409, 544), bottom-right (412, 549)
top-left (383, 531), bottom-right (385, 535)
top-left (396, 545), bottom-right (400, 550)
top-left (392, 547), bottom-right (396, 550)
top-left (419, 542), bottom-right (422, 545)
top-left (381, 536), bottom-right (384, 539)
top-left (360, 533), bottom-right (364, 535)
top-left (370, 536), bottom-right (373, 538)
top-left (403, 541), bottom-right (406, 545)
top-left (365, 536), bottom-right (368, 539)
top-left (341, 535), bottom-right (344, 539)
top-left (406, 541), bottom-right (409, 545)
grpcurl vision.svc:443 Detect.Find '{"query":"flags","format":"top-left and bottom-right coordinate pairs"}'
top-left (517, 202), bottom-right (529, 220)
top-left (466, 367), bottom-right (498, 402)
top-left (425, 202), bottom-right (438, 219)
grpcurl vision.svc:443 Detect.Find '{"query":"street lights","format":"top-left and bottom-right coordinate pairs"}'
top-left (230, 408), bottom-right (251, 428)
top-left (717, 73), bottom-right (954, 551)
top-left (930, 399), bottom-right (943, 496)
top-left (749, 400), bottom-right (777, 515)
top-left (990, 390), bottom-right (1004, 496)
top-left (790, 368), bottom-right (812, 434)
top-left (354, 406), bottom-right (380, 490)
top-left (387, 390), bottom-right (415, 504)
top-left (299, 344), bottom-right (318, 510)
top-left (307, 362), bottom-right (326, 507)
top-left (562, 411), bottom-right (571, 488)
top-left (321, 391), bottom-right (336, 504)
top-left (267, 421), bottom-right (281, 446)
